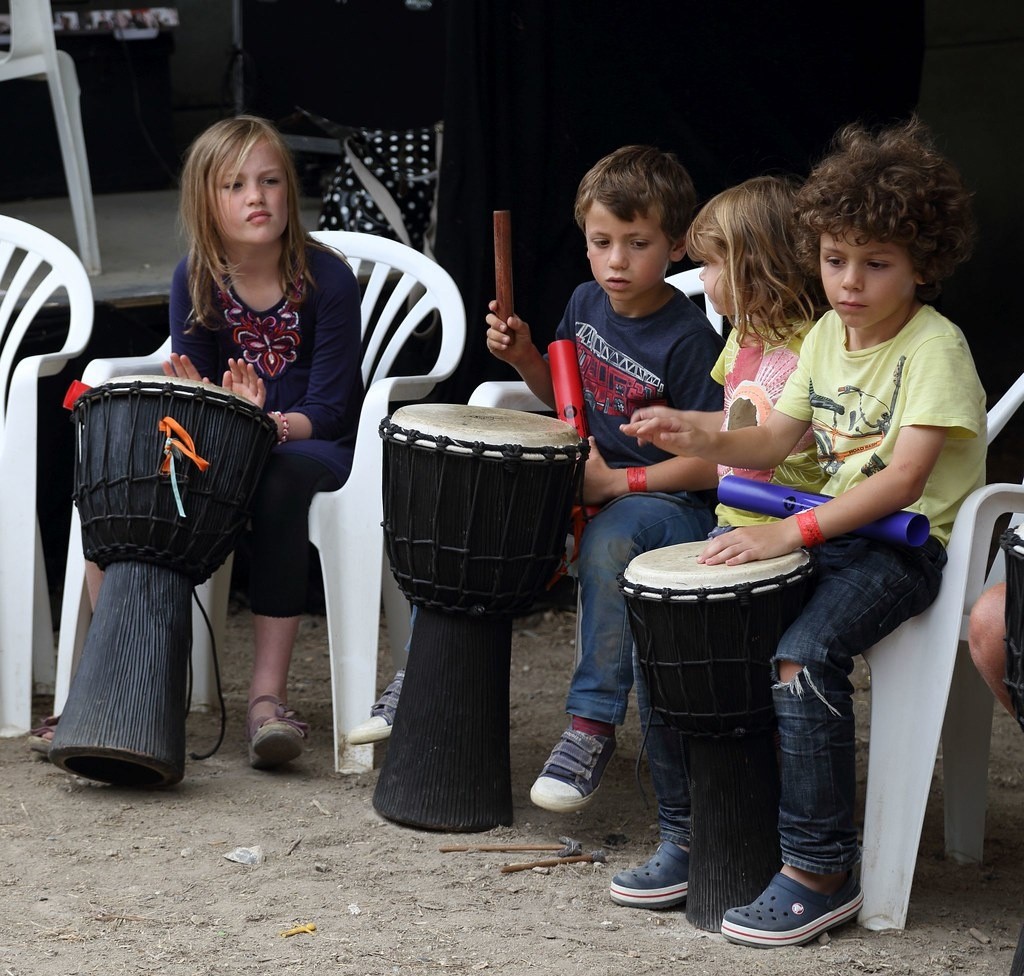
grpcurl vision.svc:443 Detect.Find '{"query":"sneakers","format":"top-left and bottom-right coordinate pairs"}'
top-left (348, 669), bottom-right (407, 744)
top-left (531, 729), bottom-right (618, 811)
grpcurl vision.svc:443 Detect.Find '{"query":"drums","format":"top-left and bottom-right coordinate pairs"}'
top-left (370, 402), bottom-right (590, 834)
top-left (619, 538), bottom-right (813, 933)
top-left (49, 375), bottom-right (277, 786)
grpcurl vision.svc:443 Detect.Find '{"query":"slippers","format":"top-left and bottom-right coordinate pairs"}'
top-left (721, 869), bottom-right (863, 950)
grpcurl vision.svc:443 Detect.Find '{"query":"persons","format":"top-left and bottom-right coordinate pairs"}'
top-left (29, 112), bottom-right (365, 770)
top-left (481, 143), bottom-right (725, 814)
top-left (580, 173), bottom-right (829, 542)
top-left (609, 112), bottom-right (989, 949)
top-left (969, 580), bottom-right (1018, 721)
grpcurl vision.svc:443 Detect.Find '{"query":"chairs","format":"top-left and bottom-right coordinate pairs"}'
top-left (854, 374), bottom-right (1024, 932)
top-left (0, 213), bottom-right (95, 740)
top-left (470, 265), bottom-right (725, 413)
top-left (0, 0), bottom-right (104, 279)
top-left (49, 228), bottom-right (469, 772)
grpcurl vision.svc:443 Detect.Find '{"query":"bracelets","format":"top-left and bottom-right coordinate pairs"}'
top-left (626, 465), bottom-right (648, 493)
top-left (795, 506), bottom-right (826, 549)
top-left (269, 410), bottom-right (290, 446)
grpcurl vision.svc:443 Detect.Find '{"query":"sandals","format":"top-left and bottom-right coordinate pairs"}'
top-left (610, 841), bottom-right (691, 908)
top-left (27, 716), bottom-right (60, 752)
top-left (246, 694), bottom-right (304, 768)
